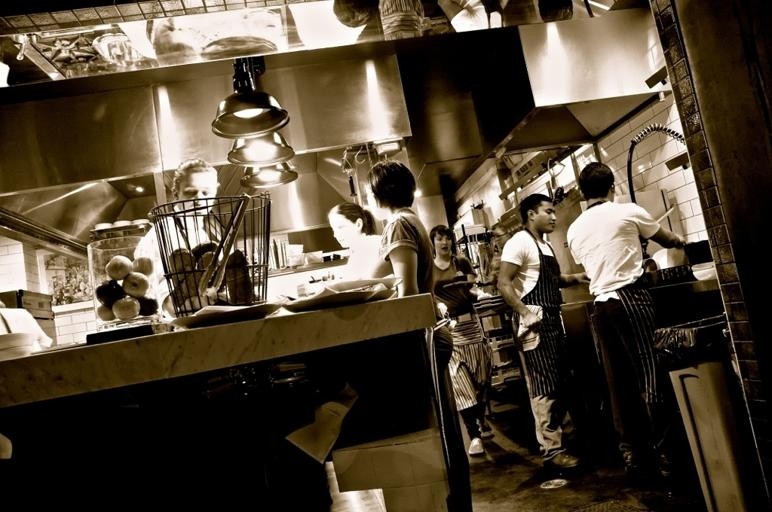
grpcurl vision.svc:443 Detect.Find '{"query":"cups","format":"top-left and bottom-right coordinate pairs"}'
top-left (88, 237), bottom-right (163, 333)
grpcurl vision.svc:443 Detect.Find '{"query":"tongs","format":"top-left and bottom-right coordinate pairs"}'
top-left (198, 193), bottom-right (252, 295)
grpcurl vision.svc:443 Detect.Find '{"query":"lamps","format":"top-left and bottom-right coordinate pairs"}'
top-left (212, 62), bottom-right (302, 191)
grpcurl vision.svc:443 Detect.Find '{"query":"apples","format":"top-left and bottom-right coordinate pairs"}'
top-left (95, 252), bottom-right (159, 322)
top-left (169, 241), bottom-right (218, 306)
top-left (153, 17), bottom-right (196, 64)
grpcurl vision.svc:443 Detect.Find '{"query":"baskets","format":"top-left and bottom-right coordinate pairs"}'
top-left (146, 192), bottom-right (272, 321)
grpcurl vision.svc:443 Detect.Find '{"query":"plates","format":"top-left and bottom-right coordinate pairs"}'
top-left (170, 290), bottom-right (397, 330)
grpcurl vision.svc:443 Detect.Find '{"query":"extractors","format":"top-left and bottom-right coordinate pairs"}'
top-left (465, 7), bottom-right (672, 163)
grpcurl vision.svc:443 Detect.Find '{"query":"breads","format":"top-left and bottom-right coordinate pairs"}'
top-left (199, 37), bottom-right (277, 59)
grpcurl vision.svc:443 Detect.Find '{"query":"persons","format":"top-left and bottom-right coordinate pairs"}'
top-left (567, 161), bottom-right (687, 475)
top-left (134, 158), bottom-right (225, 323)
top-left (497, 194), bottom-right (591, 467)
top-left (538, 0), bottom-right (573, 23)
top-left (430, 224), bottom-right (495, 455)
top-left (328, 203), bottom-right (395, 280)
top-left (366, 161), bottom-right (453, 436)
top-left (333, 0), bottom-right (510, 41)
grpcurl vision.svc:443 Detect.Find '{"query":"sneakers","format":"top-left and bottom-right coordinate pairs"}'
top-left (617, 437), bottom-right (674, 477)
top-left (544, 450), bottom-right (582, 468)
top-left (466, 424), bottom-right (495, 457)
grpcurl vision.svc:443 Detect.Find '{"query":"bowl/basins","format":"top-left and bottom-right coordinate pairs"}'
top-left (691, 262), bottom-right (717, 280)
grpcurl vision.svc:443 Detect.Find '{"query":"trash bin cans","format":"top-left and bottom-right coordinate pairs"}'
top-left (654, 314), bottom-right (734, 366)
